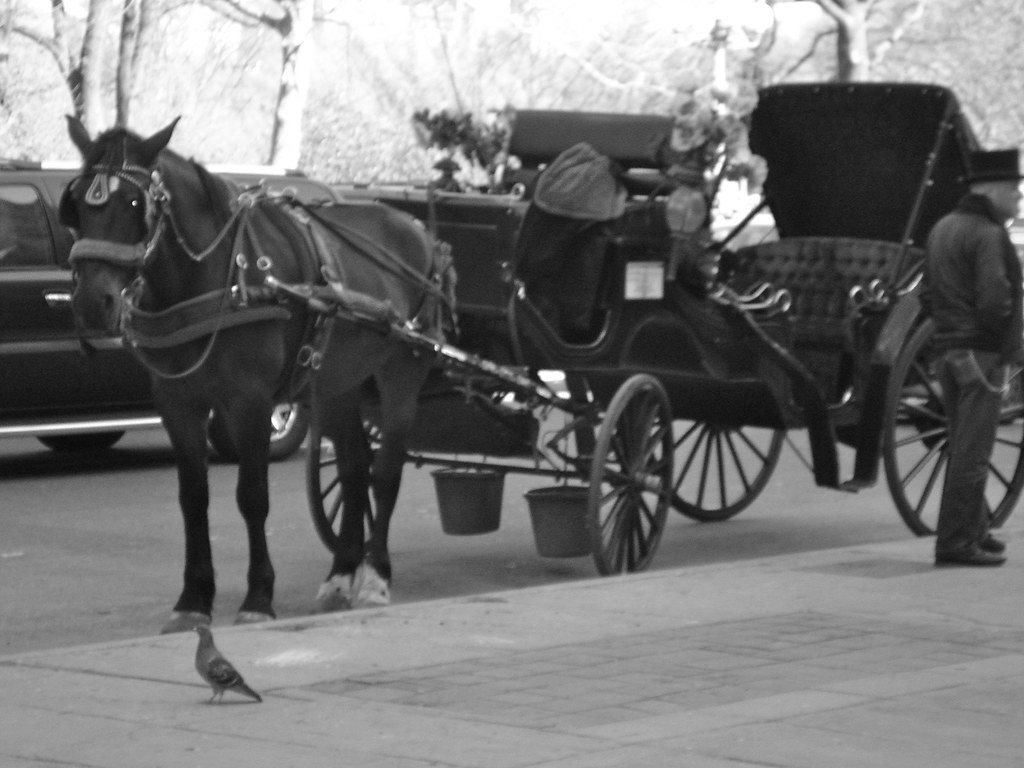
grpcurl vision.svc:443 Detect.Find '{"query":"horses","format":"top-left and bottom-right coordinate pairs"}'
top-left (59, 114), bottom-right (449, 632)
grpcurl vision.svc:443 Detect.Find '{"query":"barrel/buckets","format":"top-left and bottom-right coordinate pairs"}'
top-left (527, 484), bottom-right (602, 558)
top-left (433, 467), bottom-right (503, 536)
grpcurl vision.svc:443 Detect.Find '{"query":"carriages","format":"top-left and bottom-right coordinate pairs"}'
top-left (58, 66), bottom-right (1023, 637)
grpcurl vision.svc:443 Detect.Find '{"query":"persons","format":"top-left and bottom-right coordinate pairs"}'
top-left (918, 146), bottom-right (1024, 566)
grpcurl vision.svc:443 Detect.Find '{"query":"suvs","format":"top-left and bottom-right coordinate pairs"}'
top-left (1, 156), bottom-right (351, 470)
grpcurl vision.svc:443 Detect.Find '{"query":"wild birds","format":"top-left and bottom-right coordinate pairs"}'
top-left (192, 623), bottom-right (264, 705)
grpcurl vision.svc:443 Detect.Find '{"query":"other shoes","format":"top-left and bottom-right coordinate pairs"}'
top-left (981, 535), bottom-right (1005, 553)
top-left (935, 549), bottom-right (1006, 565)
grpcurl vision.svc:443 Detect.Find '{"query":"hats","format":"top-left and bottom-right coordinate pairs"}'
top-left (958, 149), bottom-right (1024, 182)
